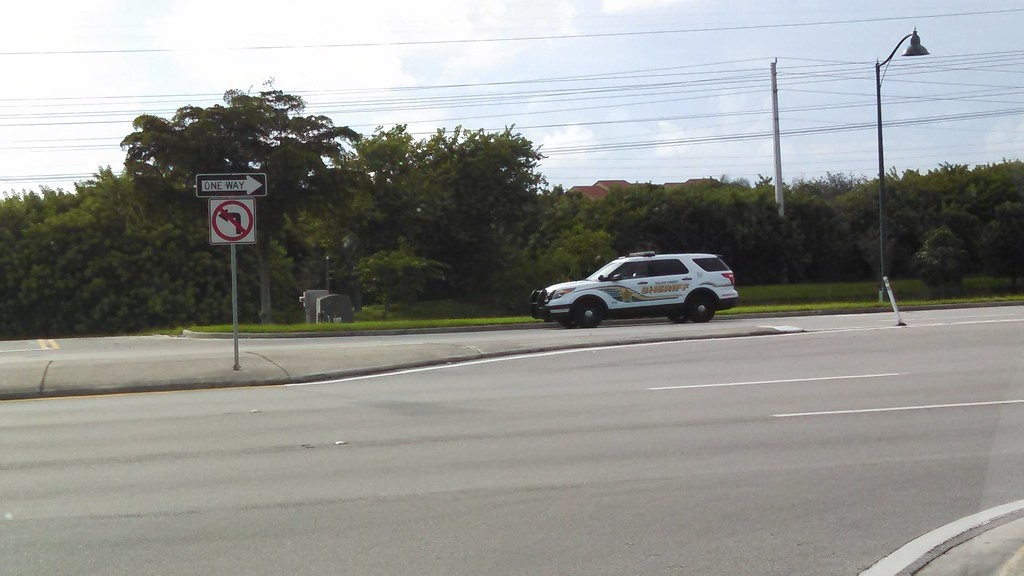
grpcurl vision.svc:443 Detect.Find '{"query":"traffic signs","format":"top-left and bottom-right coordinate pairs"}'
top-left (195, 173), bottom-right (268, 198)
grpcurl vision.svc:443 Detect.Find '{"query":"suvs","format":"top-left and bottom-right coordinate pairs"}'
top-left (528, 250), bottom-right (737, 324)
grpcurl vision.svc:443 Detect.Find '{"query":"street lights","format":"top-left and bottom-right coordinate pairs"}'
top-left (872, 27), bottom-right (929, 302)
